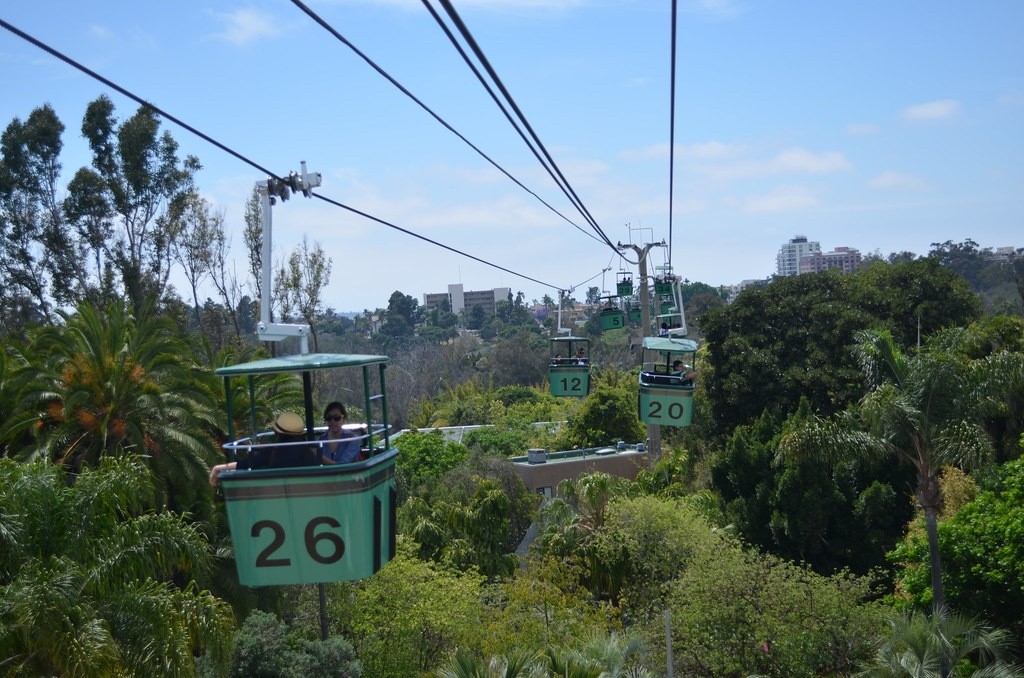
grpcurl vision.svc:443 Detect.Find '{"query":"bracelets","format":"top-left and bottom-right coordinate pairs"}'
top-left (223, 463), bottom-right (227, 470)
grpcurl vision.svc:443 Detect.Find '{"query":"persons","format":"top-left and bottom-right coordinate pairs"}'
top-left (622, 277), bottom-right (631, 283)
top-left (554, 347), bottom-right (592, 365)
top-left (659, 322), bottom-right (680, 336)
top-left (315, 401), bottom-right (362, 466)
top-left (602, 303), bottom-right (613, 311)
top-left (669, 360), bottom-right (701, 384)
top-left (209, 411), bottom-right (320, 488)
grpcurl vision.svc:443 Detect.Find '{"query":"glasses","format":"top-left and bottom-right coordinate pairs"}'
top-left (325, 415), bottom-right (344, 421)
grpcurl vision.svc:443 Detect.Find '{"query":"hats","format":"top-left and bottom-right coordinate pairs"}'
top-left (271, 412), bottom-right (305, 435)
